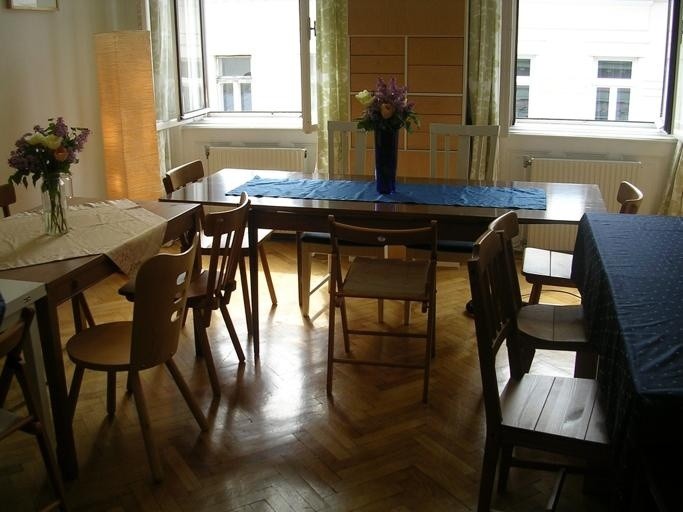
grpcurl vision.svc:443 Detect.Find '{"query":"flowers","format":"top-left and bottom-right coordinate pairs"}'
top-left (7, 115), bottom-right (93, 234)
top-left (355, 75), bottom-right (421, 135)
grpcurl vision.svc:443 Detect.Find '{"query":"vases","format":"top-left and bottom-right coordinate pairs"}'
top-left (40, 173), bottom-right (70, 236)
top-left (375, 127), bottom-right (399, 193)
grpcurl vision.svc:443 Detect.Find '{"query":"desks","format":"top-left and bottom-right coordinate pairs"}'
top-left (158, 169), bottom-right (608, 354)
top-left (570, 214), bottom-right (683, 512)
top-left (0, 195), bottom-right (205, 479)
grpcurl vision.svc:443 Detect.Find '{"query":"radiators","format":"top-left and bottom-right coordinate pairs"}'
top-left (526, 155), bottom-right (642, 253)
top-left (205, 146), bottom-right (307, 235)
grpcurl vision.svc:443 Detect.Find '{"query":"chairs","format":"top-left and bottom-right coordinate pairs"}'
top-left (163, 160), bottom-right (277, 332)
top-left (404, 122), bottom-right (500, 325)
top-left (326, 214), bottom-right (437, 403)
top-left (301, 121), bottom-right (388, 323)
top-left (118, 191), bottom-right (250, 396)
top-left (0, 183), bottom-right (69, 512)
top-left (56, 231), bottom-right (211, 483)
top-left (521, 181), bottom-right (644, 306)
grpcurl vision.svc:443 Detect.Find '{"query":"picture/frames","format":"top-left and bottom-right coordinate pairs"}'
top-left (8, 0), bottom-right (60, 12)
